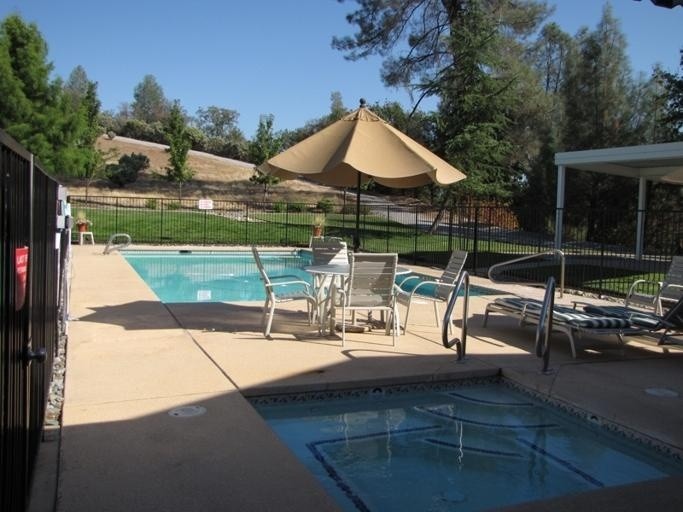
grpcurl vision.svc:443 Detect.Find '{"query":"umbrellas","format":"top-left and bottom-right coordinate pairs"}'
top-left (253, 96), bottom-right (467, 254)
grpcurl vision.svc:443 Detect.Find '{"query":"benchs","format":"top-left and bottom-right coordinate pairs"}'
top-left (483, 295), bottom-right (648, 362)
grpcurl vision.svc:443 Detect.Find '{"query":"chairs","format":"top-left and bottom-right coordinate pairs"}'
top-left (584, 287), bottom-right (681, 347)
top-left (247, 234), bottom-right (470, 347)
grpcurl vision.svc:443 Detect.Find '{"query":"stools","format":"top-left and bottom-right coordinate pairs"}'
top-left (71, 231), bottom-right (95, 247)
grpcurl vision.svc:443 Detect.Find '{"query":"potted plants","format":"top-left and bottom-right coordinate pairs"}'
top-left (311, 214), bottom-right (326, 235)
top-left (74, 209), bottom-right (90, 231)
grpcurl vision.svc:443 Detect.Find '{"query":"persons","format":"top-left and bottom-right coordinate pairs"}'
top-left (313, 203), bottom-right (326, 237)
